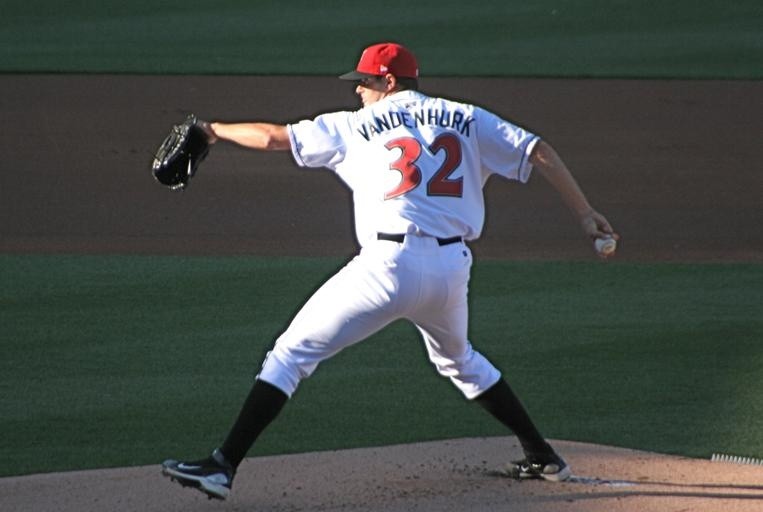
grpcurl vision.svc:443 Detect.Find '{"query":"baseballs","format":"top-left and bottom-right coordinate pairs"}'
top-left (594, 234), bottom-right (616, 253)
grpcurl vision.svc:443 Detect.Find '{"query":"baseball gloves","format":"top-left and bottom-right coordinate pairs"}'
top-left (151, 122), bottom-right (211, 191)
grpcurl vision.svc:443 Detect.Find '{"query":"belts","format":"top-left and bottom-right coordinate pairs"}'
top-left (378, 234), bottom-right (461, 246)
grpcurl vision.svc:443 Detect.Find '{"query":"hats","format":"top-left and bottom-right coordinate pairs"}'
top-left (339, 43), bottom-right (419, 81)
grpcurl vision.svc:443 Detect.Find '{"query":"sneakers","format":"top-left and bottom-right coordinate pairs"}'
top-left (163, 448), bottom-right (236, 500)
top-left (507, 450), bottom-right (570, 482)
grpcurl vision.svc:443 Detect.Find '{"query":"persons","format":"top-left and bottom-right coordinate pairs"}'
top-left (150, 42), bottom-right (622, 502)
